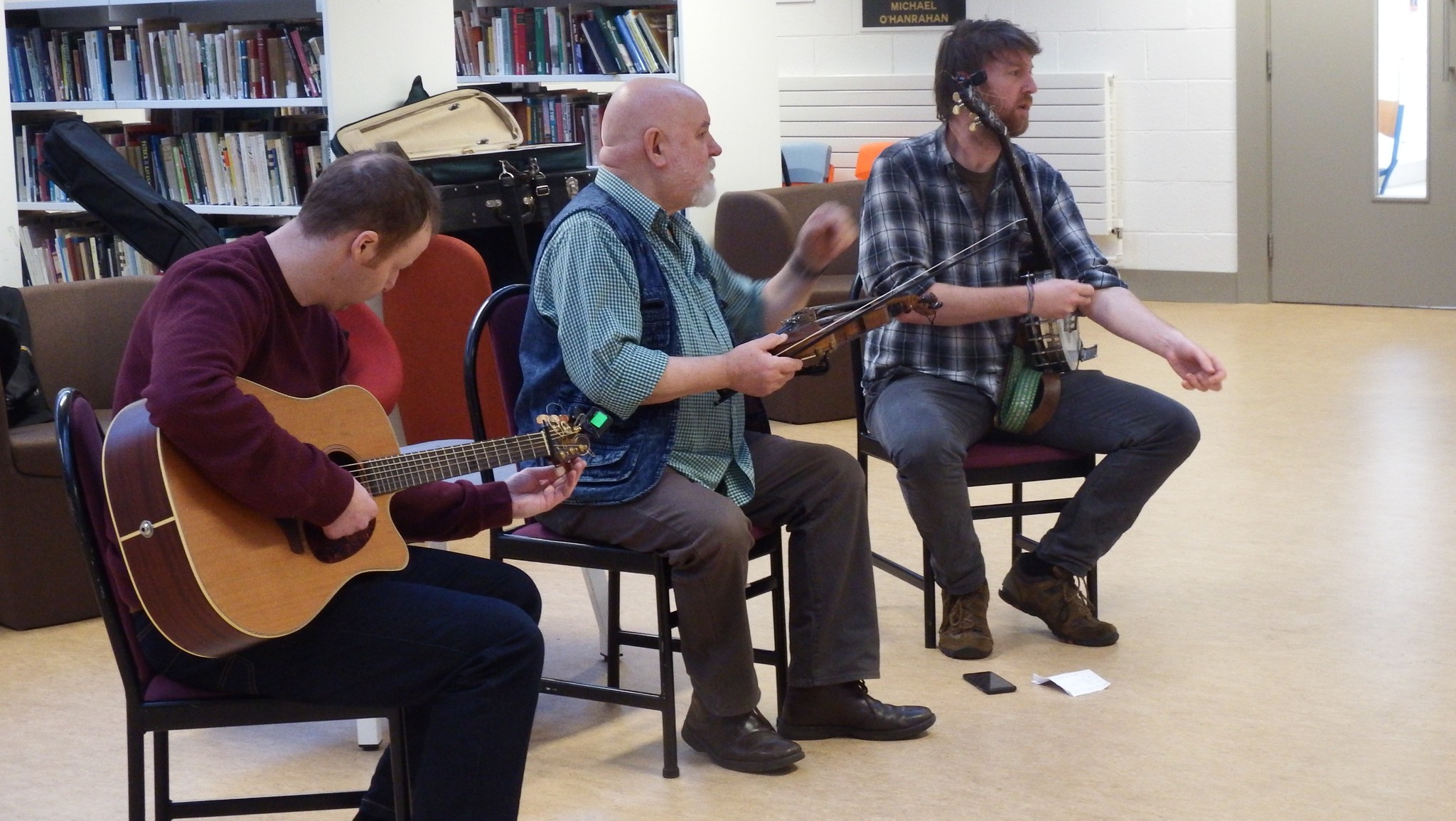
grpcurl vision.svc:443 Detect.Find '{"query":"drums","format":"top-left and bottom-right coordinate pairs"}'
top-left (1019, 270), bottom-right (1081, 374)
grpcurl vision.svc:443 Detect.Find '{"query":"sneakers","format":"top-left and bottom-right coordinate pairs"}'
top-left (938, 578), bottom-right (994, 660)
top-left (998, 552), bottom-right (1119, 647)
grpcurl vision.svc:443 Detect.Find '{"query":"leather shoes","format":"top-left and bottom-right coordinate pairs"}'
top-left (682, 706), bottom-right (805, 772)
top-left (776, 679), bottom-right (936, 741)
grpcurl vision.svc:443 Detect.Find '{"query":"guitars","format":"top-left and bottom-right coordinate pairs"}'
top-left (102, 376), bottom-right (594, 660)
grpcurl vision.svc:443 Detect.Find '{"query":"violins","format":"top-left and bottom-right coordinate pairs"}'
top-left (766, 291), bottom-right (945, 369)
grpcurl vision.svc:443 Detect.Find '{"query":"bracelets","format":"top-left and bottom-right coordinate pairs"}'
top-left (787, 252), bottom-right (824, 280)
top-left (1022, 281), bottom-right (1034, 322)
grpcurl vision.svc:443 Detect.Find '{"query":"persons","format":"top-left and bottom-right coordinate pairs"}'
top-left (110, 150), bottom-right (587, 821)
top-left (512, 73), bottom-right (937, 775)
top-left (858, 17), bottom-right (1226, 660)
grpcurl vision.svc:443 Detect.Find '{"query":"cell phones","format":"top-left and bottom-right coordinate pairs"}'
top-left (963, 670), bottom-right (1017, 695)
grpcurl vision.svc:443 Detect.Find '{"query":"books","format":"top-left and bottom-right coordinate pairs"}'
top-left (454, 0), bottom-right (680, 167)
top-left (8, 11), bottom-right (331, 287)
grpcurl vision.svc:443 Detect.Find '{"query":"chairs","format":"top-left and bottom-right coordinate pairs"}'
top-left (0, 138), bottom-right (1102, 821)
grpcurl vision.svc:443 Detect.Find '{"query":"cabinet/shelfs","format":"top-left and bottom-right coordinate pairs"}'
top-left (0, 0), bottom-right (455, 287)
top-left (452, 0), bottom-right (781, 248)
